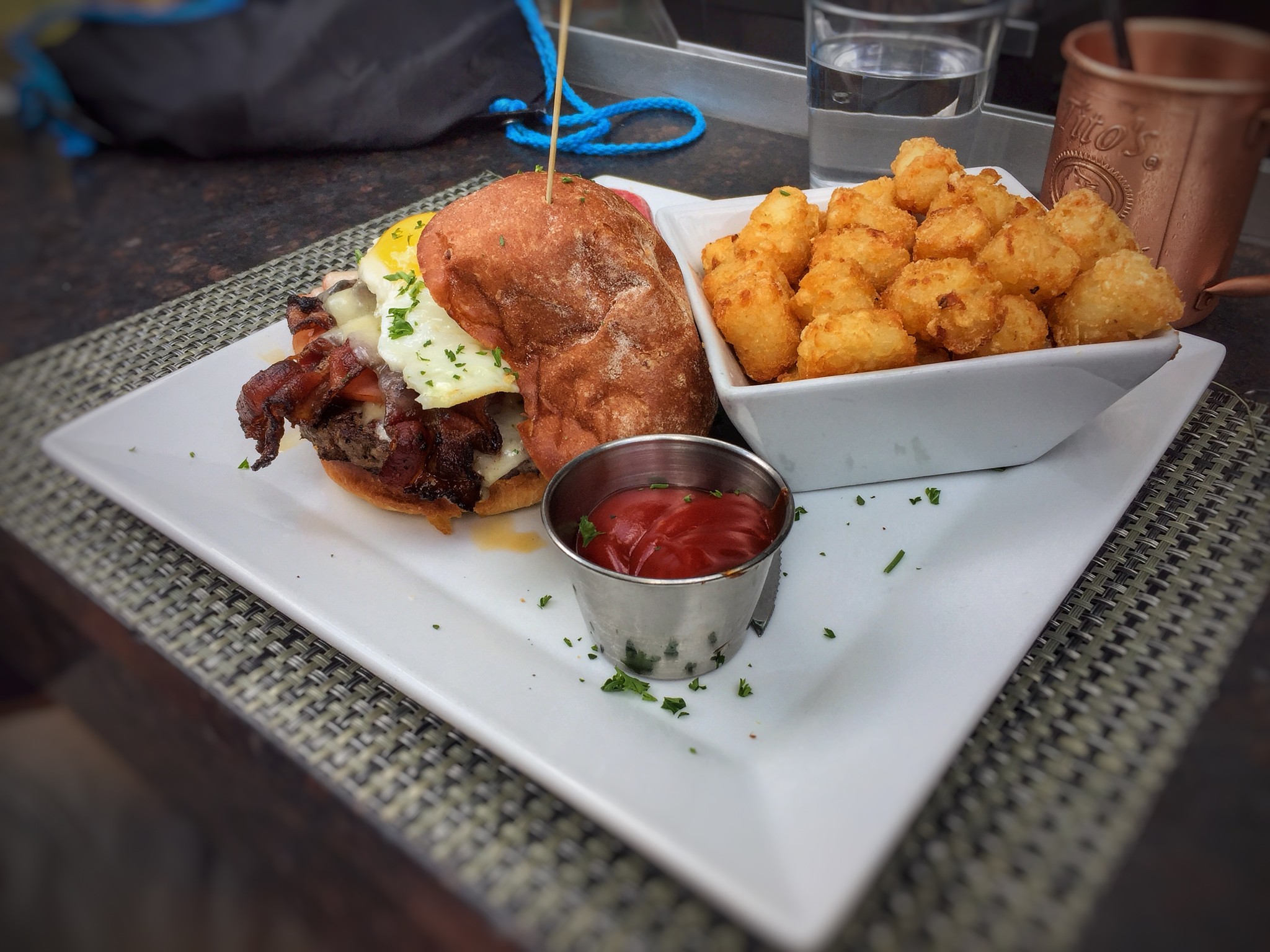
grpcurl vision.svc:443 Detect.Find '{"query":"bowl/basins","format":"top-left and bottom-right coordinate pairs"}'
top-left (650, 165), bottom-right (1185, 492)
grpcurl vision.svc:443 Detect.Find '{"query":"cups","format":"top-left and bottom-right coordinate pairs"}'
top-left (540, 433), bottom-right (796, 681)
top-left (1039, 19), bottom-right (1270, 330)
top-left (800, 0), bottom-right (1020, 192)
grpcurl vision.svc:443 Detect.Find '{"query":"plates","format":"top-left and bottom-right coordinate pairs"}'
top-left (35, 174), bottom-right (1229, 952)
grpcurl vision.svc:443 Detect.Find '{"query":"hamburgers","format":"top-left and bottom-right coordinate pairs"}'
top-left (234, 171), bottom-right (721, 536)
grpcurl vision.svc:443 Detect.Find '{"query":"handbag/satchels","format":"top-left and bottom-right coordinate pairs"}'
top-left (8, 0), bottom-right (706, 153)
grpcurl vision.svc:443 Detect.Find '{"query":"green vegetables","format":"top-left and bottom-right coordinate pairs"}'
top-left (540, 470), bottom-right (940, 716)
